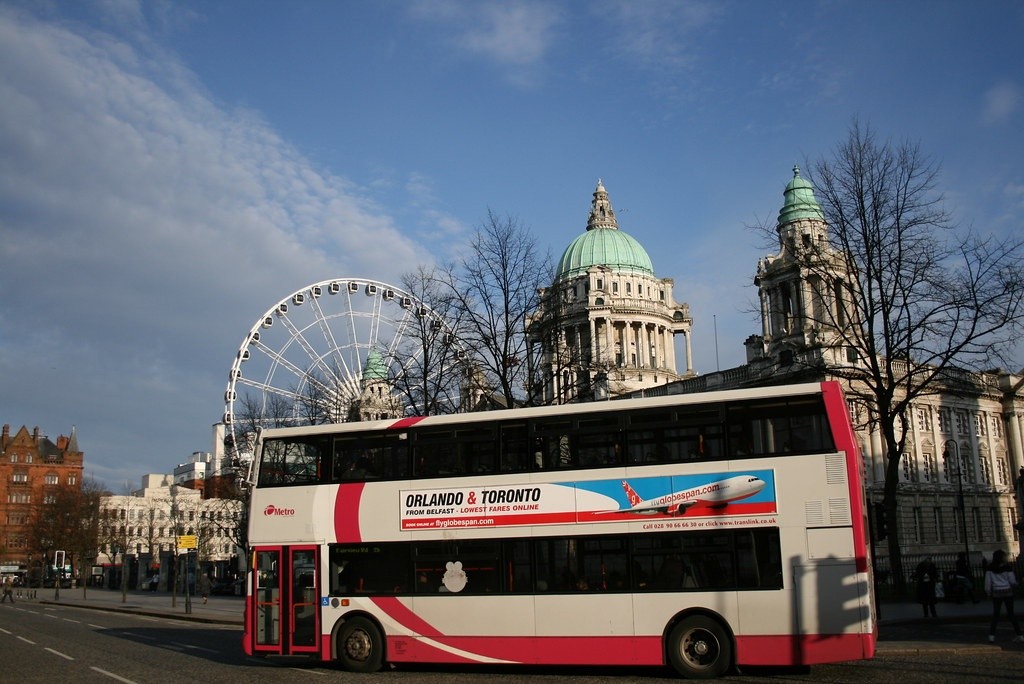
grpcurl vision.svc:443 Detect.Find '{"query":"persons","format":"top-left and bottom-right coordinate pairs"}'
top-left (916, 557), bottom-right (939, 618)
top-left (200, 573), bottom-right (214, 604)
top-left (0, 576), bottom-right (14, 603)
top-left (985, 549), bottom-right (1024, 642)
top-left (151, 571), bottom-right (159, 593)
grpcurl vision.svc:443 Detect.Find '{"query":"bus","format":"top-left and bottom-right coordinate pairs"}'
top-left (242, 384), bottom-right (877, 678)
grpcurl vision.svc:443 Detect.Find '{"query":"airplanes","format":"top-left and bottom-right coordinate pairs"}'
top-left (590, 474), bottom-right (765, 519)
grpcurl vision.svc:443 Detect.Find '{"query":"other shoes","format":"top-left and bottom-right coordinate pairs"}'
top-left (1012, 635), bottom-right (1024, 643)
top-left (988, 635), bottom-right (995, 642)
